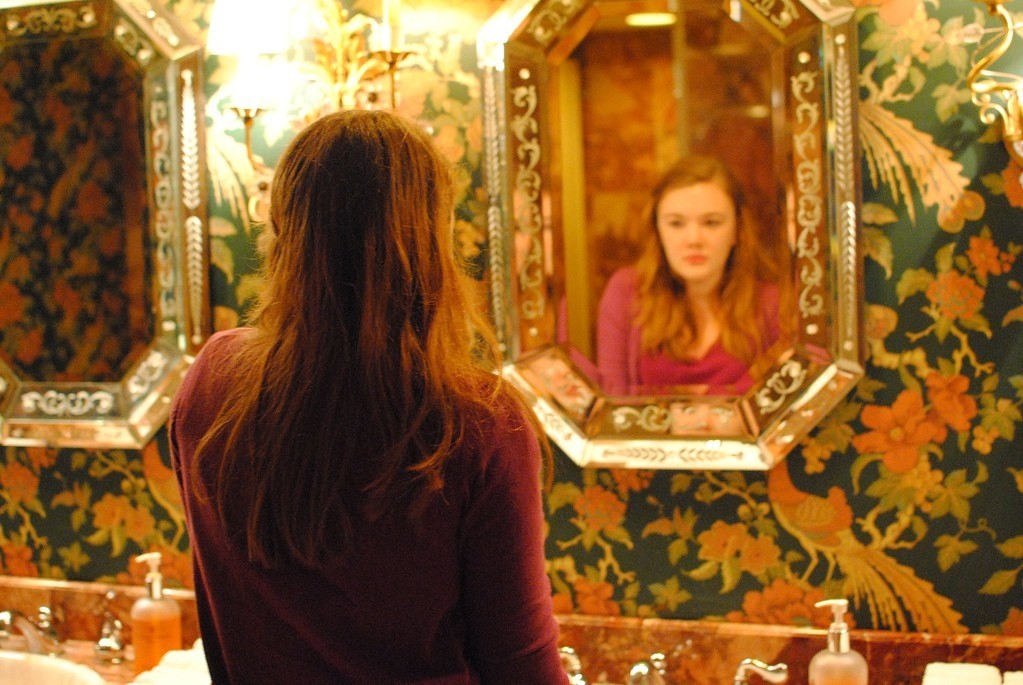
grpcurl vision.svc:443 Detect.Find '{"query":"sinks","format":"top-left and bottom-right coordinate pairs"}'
top-left (0, 650), bottom-right (108, 685)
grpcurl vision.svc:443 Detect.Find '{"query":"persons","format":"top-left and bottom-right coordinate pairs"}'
top-left (595, 153), bottom-right (802, 400)
top-left (170, 113), bottom-right (574, 685)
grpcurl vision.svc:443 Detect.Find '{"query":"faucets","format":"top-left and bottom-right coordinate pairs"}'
top-left (626, 650), bottom-right (667, 683)
top-left (1, 606), bottom-right (62, 656)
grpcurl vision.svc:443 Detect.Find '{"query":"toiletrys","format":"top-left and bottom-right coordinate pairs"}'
top-left (806, 599), bottom-right (869, 684)
top-left (130, 551), bottom-right (182, 677)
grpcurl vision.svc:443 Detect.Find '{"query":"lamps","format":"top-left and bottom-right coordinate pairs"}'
top-left (206, 2), bottom-right (414, 172)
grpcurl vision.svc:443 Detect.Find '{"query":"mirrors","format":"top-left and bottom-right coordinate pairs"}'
top-left (1, 3), bottom-right (207, 451)
top-left (477, 0), bottom-right (864, 471)
